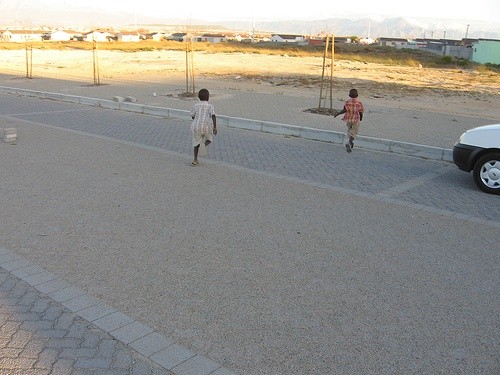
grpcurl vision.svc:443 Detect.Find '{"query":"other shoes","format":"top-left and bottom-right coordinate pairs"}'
top-left (346, 142), bottom-right (354, 152)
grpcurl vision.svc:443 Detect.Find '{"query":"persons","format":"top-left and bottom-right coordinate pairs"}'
top-left (334, 88), bottom-right (365, 152)
top-left (191, 88), bottom-right (218, 166)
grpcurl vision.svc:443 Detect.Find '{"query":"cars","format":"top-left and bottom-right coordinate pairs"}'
top-left (452, 123), bottom-right (500, 195)
